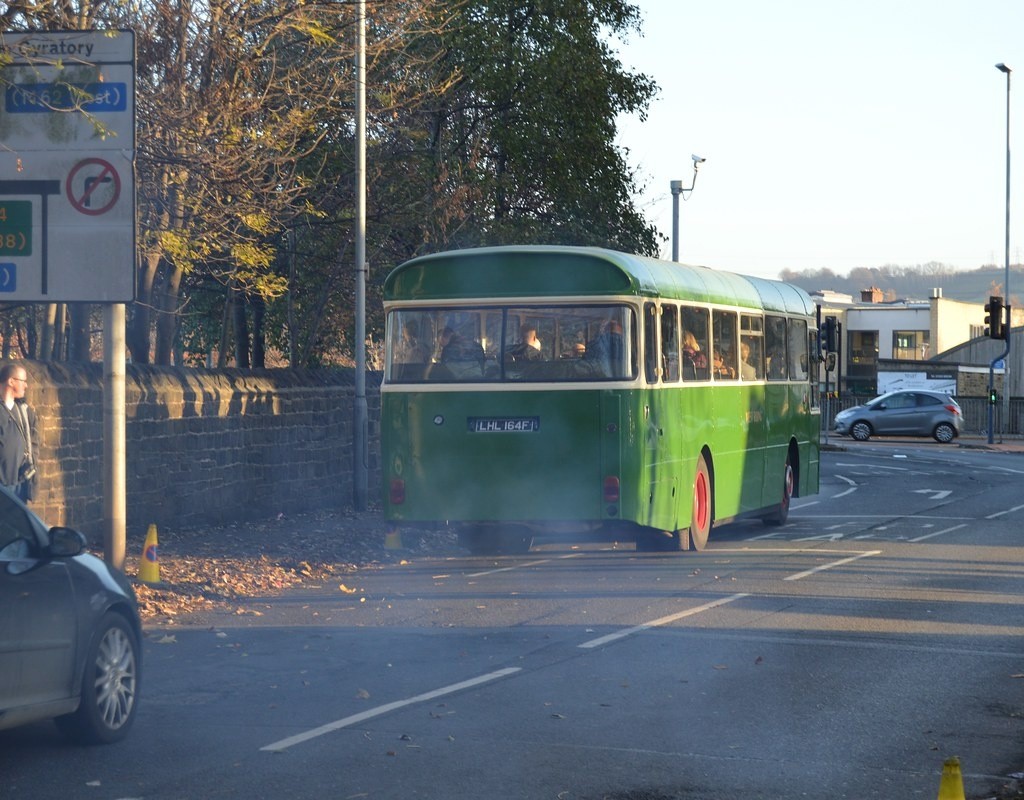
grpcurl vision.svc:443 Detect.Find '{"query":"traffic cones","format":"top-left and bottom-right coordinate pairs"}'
top-left (129, 523), bottom-right (170, 589)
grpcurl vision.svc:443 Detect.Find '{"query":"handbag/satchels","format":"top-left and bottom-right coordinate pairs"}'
top-left (24, 467), bottom-right (39, 501)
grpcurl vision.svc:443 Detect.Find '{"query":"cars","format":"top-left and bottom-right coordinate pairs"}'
top-left (0, 481), bottom-right (143, 745)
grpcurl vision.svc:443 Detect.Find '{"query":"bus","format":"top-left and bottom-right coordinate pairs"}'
top-left (381, 246), bottom-right (836, 551)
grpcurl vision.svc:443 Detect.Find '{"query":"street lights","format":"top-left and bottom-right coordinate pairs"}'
top-left (995, 62), bottom-right (1011, 437)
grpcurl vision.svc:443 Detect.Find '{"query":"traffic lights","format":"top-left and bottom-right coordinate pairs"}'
top-left (821, 315), bottom-right (836, 352)
top-left (984, 295), bottom-right (1003, 339)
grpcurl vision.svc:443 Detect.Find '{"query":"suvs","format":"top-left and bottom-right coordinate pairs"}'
top-left (832, 389), bottom-right (964, 443)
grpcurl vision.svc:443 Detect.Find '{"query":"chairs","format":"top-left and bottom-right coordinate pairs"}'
top-left (484, 354), bottom-right (722, 380)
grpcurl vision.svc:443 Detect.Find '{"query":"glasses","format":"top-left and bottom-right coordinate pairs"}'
top-left (11, 378), bottom-right (28, 383)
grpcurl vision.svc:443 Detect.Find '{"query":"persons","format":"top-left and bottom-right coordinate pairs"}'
top-left (682, 332), bottom-right (708, 367)
top-left (506, 324), bottom-right (546, 360)
top-left (436, 328), bottom-right (485, 371)
top-left (741, 343), bottom-right (756, 380)
top-left (0, 363), bottom-right (40, 505)
top-left (582, 320), bottom-right (635, 359)
top-left (714, 345), bottom-right (735, 377)
